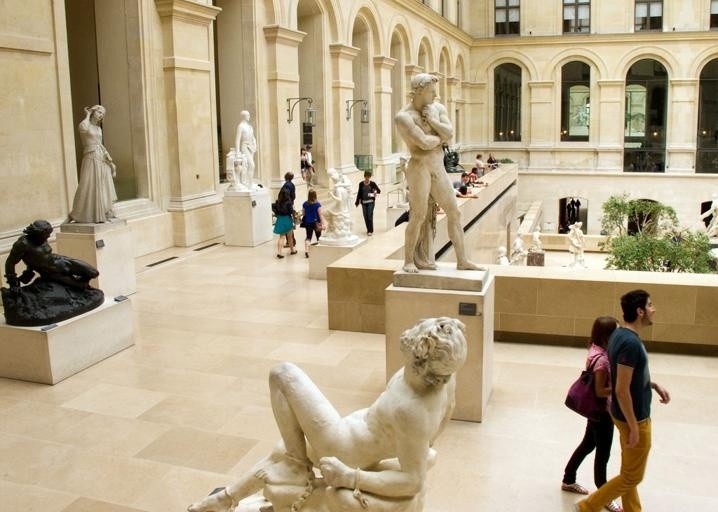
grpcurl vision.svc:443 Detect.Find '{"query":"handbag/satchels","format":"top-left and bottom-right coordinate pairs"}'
top-left (313, 222), bottom-right (327, 231)
top-left (564, 353), bottom-right (609, 424)
top-left (300, 215), bottom-right (306, 228)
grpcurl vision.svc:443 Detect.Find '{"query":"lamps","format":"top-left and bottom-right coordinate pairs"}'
top-left (288, 96), bottom-right (316, 128)
top-left (345, 99), bottom-right (370, 124)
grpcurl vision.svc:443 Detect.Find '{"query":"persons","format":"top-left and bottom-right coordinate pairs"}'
top-left (5, 219), bottom-right (99, 296)
top-left (451, 152), bottom-right (499, 198)
top-left (325, 167), bottom-right (353, 241)
top-left (273, 172), bottom-right (298, 258)
top-left (495, 223), bottom-right (543, 265)
top-left (355, 170), bottom-right (381, 236)
top-left (301, 145), bottom-right (316, 187)
top-left (302, 190), bottom-right (327, 258)
top-left (568, 221), bottom-right (585, 265)
top-left (394, 72), bottom-right (487, 273)
top-left (62, 105), bottom-right (118, 225)
top-left (566, 197), bottom-right (581, 220)
top-left (187, 316), bottom-right (468, 512)
top-left (561, 289), bottom-right (670, 512)
top-left (235, 110), bottom-right (257, 185)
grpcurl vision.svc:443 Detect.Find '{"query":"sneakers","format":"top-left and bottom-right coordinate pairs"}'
top-left (560, 482), bottom-right (589, 496)
top-left (569, 503), bottom-right (581, 512)
top-left (276, 250), bottom-right (309, 258)
top-left (605, 499), bottom-right (624, 512)
top-left (368, 232), bottom-right (373, 236)
